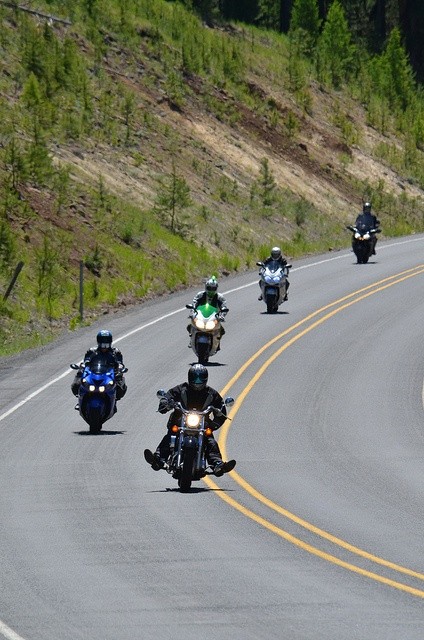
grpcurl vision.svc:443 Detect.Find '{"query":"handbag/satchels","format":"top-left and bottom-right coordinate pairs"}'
top-left (117, 376), bottom-right (127, 398)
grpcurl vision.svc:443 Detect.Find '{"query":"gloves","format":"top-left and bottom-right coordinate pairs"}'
top-left (218, 315), bottom-right (225, 322)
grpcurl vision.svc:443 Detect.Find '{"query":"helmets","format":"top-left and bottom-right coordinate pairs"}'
top-left (271, 247), bottom-right (281, 260)
top-left (97, 331), bottom-right (112, 352)
top-left (363, 203), bottom-right (371, 213)
top-left (206, 280), bottom-right (218, 297)
top-left (188, 364), bottom-right (208, 392)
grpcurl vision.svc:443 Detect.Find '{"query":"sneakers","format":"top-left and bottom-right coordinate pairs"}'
top-left (258, 295), bottom-right (262, 300)
top-left (284, 295), bottom-right (288, 301)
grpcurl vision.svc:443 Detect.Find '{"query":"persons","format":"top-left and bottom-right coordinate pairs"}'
top-left (258, 247), bottom-right (290, 301)
top-left (71, 330), bottom-right (127, 399)
top-left (186, 279), bottom-right (228, 340)
top-left (356, 202), bottom-right (380, 254)
top-left (145, 364), bottom-right (236, 476)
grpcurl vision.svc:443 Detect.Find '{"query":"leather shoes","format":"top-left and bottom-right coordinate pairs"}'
top-left (214, 460), bottom-right (236, 477)
top-left (144, 449), bottom-right (162, 471)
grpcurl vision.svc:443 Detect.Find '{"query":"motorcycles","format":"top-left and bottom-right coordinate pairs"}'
top-left (69, 357), bottom-right (127, 432)
top-left (255, 262), bottom-right (292, 313)
top-left (347, 220), bottom-right (379, 264)
top-left (185, 304), bottom-right (229, 364)
top-left (155, 390), bottom-right (234, 491)
